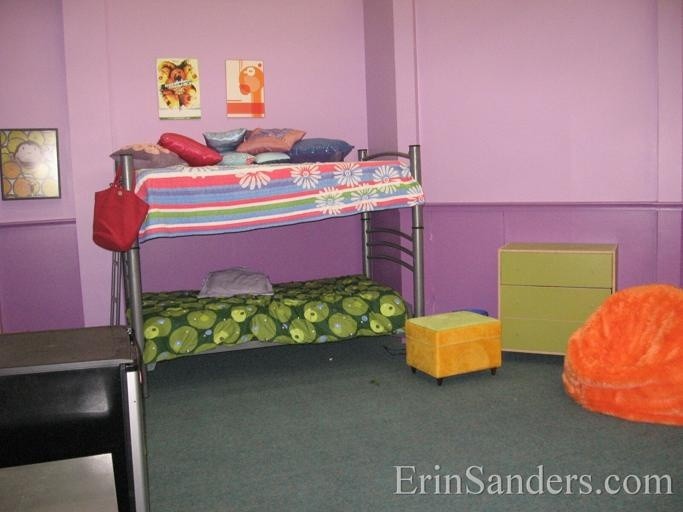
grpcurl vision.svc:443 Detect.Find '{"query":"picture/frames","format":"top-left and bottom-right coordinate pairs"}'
top-left (0, 127), bottom-right (60, 201)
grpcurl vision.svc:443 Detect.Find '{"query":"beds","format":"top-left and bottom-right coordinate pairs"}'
top-left (104, 144), bottom-right (426, 397)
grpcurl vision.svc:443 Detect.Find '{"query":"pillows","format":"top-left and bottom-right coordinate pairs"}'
top-left (106, 129), bottom-right (350, 166)
top-left (197, 267), bottom-right (273, 298)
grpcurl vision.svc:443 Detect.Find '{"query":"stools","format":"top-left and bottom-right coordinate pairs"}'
top-left (404, 309), bottom-right (504, 384)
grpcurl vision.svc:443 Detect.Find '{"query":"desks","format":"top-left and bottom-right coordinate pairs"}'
top-left (0, 326), bottom-right (150, 512)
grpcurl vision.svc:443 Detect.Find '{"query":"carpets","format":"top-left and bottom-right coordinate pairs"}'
top-left (137, 333), bottom-right (683, 509)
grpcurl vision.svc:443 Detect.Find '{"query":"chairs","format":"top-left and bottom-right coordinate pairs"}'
top-left (561, 283), bottom-right (683, 426)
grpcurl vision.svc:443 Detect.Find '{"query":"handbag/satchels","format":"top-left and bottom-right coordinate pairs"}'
top-left (94, 165), bottom-right (148, 253)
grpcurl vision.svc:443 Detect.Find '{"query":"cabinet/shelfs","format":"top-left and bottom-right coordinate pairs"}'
top-left (495, 241), bottom-right (618, 358)
